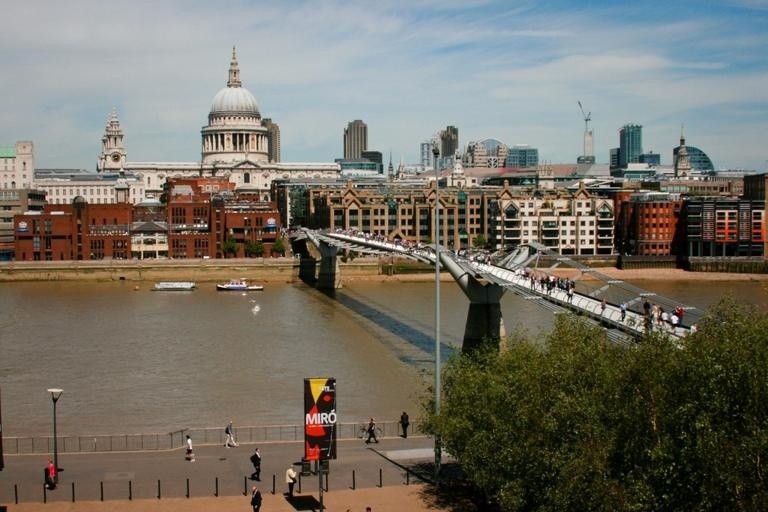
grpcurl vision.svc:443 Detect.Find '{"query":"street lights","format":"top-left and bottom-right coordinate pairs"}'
top-left (47, 388), bottom-right (63, 483)
top-left (431, 138), bottom-right (443, 476)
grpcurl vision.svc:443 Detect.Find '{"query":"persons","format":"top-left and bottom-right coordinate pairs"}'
top-left (365, 417), bottom-right (379, 443)
top-left (251, 485), bottom-right (262, 512)
top-left (251, 447), bottom-right (263, 481)
top-left (468, 252), bottom-right (491, 267)
top-left (600, 297), bottom-right (606, 309)
top-left (366, 506), bottom-right (371, 511)
top-left (619, 300), bottom-right (626, 321)
top-left (640, 294), bottom-right (685, 330)
top-left (690, 322), bottom-right (697, 334)
top-left (285, 464), bottom-right (297, 496)
top-left (401, 411), bottom-right (410, 438)
top-left (334, 226), bottom-right (424, 255)
top-left (513, 267), bottom-right (577, 303)
top-left (48, 458), bottom-right (55, 481)
top-left (185, 433), bottom-right (195, 461)
top-left (225, 420), bottom-right (237, 448)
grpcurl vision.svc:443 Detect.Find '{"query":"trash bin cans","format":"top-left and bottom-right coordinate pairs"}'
top-left (319, 460), bottom-right (330, 474)
top-left (302, 457), bottom-right (311, 476)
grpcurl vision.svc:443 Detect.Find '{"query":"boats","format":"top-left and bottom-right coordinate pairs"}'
top-left (152, 281), bottom-right (195, 291)
top-left (216, 281), bottom-right (264, 291)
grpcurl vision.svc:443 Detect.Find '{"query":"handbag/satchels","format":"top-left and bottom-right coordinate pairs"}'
top-left (250, 455), bottom-right (255, 463)
top-left (289, 475), bottom-right (297, 483)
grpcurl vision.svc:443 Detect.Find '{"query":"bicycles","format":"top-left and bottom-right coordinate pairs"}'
top-left (356, 422), bottom-right (382, 439)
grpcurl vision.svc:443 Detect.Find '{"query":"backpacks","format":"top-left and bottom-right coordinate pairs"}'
top-left (225, 427), bottom-right (230, 434)
